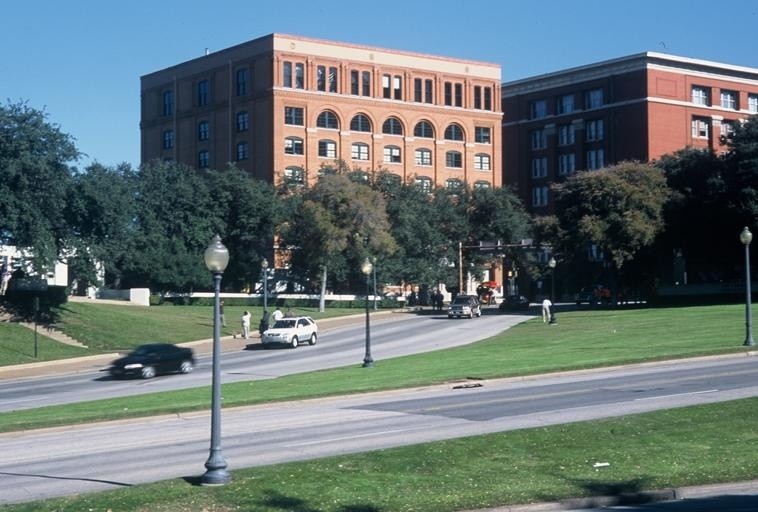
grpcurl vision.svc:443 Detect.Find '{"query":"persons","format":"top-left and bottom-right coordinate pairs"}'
top-left (260, 311), bottom-right (269, 338)
top-left (542, 298), bottom-right (552, 323)
top-left (241, 311), bottom-right (251, 339)
top-left (431, 292), bottom-right (437, 311)
top-left (219, 301), bottom-right (226, 327)
top-left (285, 308), bottom-right (294, 318)
top-left (477, 287), bottom-right (494, 305)
top-left (272, 308), bottom-right (283, 321)
top-left (435, 291), bottom-right (443, 311)
top-left (592, 288), bottom-right (624, 307)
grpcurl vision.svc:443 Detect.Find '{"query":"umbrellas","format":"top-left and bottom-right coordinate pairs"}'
top-left (479, 281), bottom-right (498, 288)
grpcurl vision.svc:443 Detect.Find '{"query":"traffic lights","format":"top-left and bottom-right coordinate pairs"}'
top-left (507, 271), bottom-right (515, 277)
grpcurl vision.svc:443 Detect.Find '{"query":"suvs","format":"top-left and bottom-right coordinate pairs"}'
top-left (446, 293), bottom-right (482, 320)
top-left (572, 284), bottom-right (623, 307)
top-left (259, 314), bottom-right (320, 351)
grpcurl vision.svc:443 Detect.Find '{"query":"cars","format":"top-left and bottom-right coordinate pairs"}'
top-left (106, 342), bottom-right (197, 382)
top-left (498, 295), bottom-right (529, 310)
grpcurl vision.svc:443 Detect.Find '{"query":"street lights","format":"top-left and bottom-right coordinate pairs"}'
top-left (371, 254), bottom-right (377, 312)
top-left (738, 224), bottom-right (756, 346)
top-left (260, 257), bottom-right (269, 314)
top-left (358, 256), bottom-right (375, 369)
top-left (547, 255), bottom-right (557, 310)
top-left (197, 232), bottom-right (234, 489)
